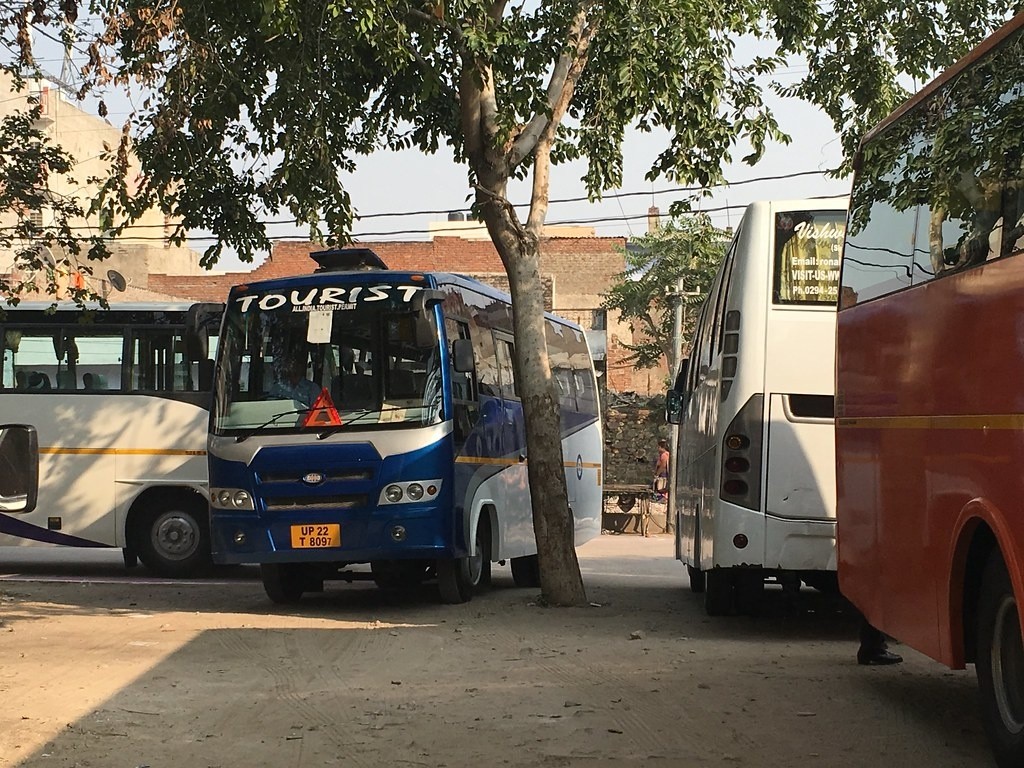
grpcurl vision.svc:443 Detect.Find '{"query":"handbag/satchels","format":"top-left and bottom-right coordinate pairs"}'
top-left (657, 477), bottom-right (666, 490)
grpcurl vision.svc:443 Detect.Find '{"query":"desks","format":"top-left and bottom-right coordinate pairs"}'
top-left (602, 482), bottom-right (651, 537)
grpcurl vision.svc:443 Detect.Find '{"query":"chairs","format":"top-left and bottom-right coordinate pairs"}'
top-left (330, 363), bottom-right (436, 403)
top-left (18, 367), bottom-right (108, 389)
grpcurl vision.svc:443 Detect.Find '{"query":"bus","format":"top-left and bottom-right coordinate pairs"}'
top-left (834, 0), bottom-right (1024, 768)
top-left (0, 292), bottom-right (228, 580)
top-left (207, 247), bottom-right (605, 608)
top-left (665, 200), bottom-right (848, 619)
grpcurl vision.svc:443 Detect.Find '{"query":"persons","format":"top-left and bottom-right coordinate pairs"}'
top-left (268, 354), bottom-right (321, 409)
top-left (653, 442), bottom-right (670, 503)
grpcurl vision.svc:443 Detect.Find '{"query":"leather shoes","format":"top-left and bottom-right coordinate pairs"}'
top-left (857, 646), bottom-right (903, 665)
